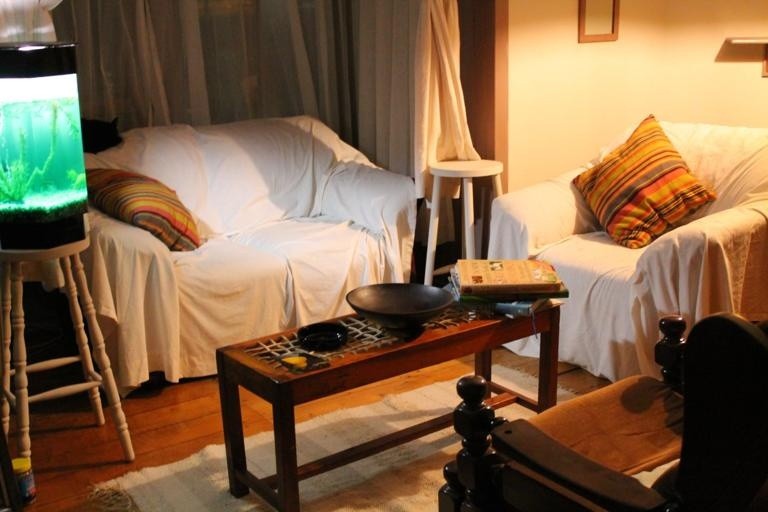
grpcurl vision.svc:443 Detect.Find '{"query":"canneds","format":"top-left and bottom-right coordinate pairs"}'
top-left (11, 457), bottom-right (38, 506)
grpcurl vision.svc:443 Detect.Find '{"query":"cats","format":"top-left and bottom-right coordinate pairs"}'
top-left (81, 116), bottom-right (122, 153)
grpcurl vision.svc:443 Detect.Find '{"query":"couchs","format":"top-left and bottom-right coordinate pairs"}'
top-left (84, 115), bottom-right (419, 400)
top-left (488, 122), bottom-right (766, 384)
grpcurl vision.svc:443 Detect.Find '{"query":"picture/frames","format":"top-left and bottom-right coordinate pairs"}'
top-left (578, 0), bottom-right (619, 44)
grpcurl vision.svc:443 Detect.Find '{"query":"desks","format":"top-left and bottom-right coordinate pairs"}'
top-left (214, 288), bottom-right (560, 511)
top-left (2, 236), bottom-right (137, 465)
top-left (426, 160), bottom-right (505, 286)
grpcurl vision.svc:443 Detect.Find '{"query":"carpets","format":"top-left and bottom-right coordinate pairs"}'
top-left (75, 364), bottom-right (579, 511)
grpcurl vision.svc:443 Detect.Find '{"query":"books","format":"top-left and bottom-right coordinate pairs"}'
top-left (441, 259), bottom-right (570, 318)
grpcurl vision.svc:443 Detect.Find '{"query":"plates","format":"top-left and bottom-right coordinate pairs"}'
top-left (346, 283), bottom-right (454, 330)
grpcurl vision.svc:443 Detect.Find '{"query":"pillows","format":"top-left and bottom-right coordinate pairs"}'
top-left (84, 164), bottom-right (201, 252)
top-left (573, 110), bottom-right (716, 250)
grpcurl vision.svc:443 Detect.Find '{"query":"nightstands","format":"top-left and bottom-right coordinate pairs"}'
top-left (438, 310), bottom-right (767, 510)
